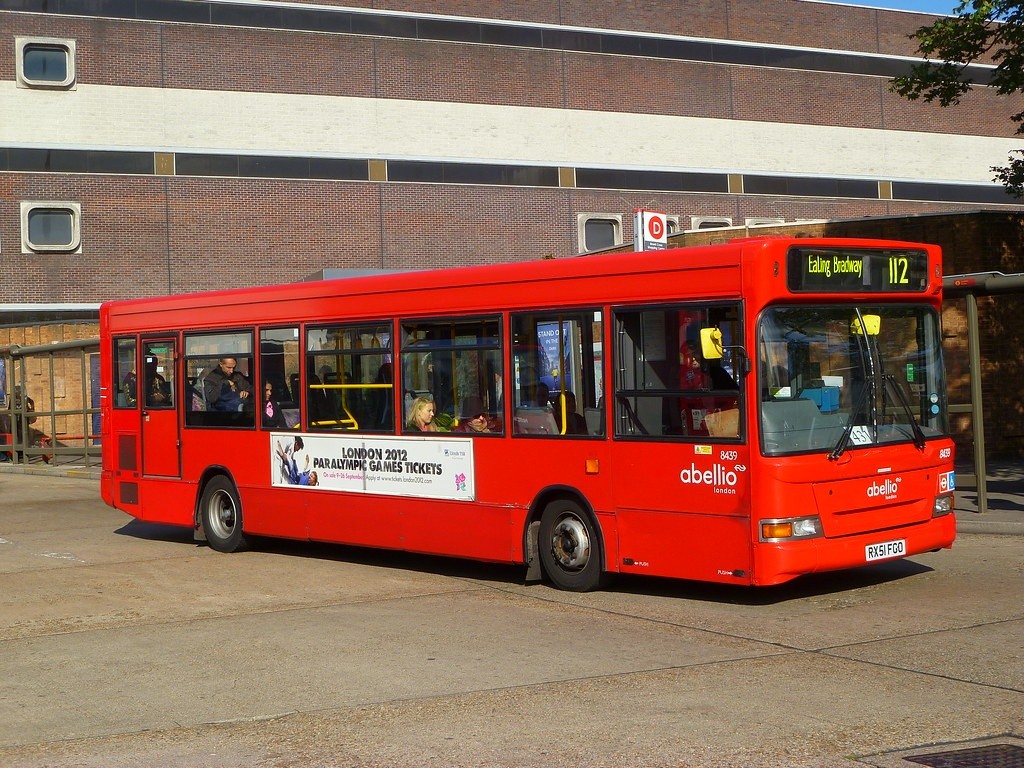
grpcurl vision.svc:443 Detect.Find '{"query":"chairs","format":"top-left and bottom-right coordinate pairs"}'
top-left (123, 361), bottom-right (605, 434)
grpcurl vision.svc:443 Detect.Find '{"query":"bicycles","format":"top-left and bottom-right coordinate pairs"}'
top-left (551, 373), bottom-right (559, 387)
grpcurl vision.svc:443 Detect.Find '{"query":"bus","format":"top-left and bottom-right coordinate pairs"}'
top-left (100, 236), bottom-right (957, 592)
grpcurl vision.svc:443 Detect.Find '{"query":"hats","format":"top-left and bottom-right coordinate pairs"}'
top-left (457, 396), bottom-right (489, 418)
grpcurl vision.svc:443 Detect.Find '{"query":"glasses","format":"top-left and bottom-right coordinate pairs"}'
top-left (473, 413), bottom-right (487, 420)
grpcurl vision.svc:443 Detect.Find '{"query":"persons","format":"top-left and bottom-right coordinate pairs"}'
top-left (521, 366), bottom-right (552, 415)
top-left (597, 377), bottom-right (603, 408)
top-left (552, 368), bottom-right (559, 387)
top-left (487, 368), bottom-right (502, 409)
top-left (552, 391), bottom-right (588, 435)
top-left (451, 395), bottom-right (495, 432)
top-left (9, 384), bottom-right (34, 460)
top-left (123, 352), bottom-right (165, 406)
top-left (680, 341), bottom-right (738, 390)
top-left (406, 397), bottom-right (438, 432)
top-left (243, 379), bottom-right (287, 427)
top-left (275, 436), bottom-right (317, 485)
top-left (204, 357), bottom-right (253, 411)
